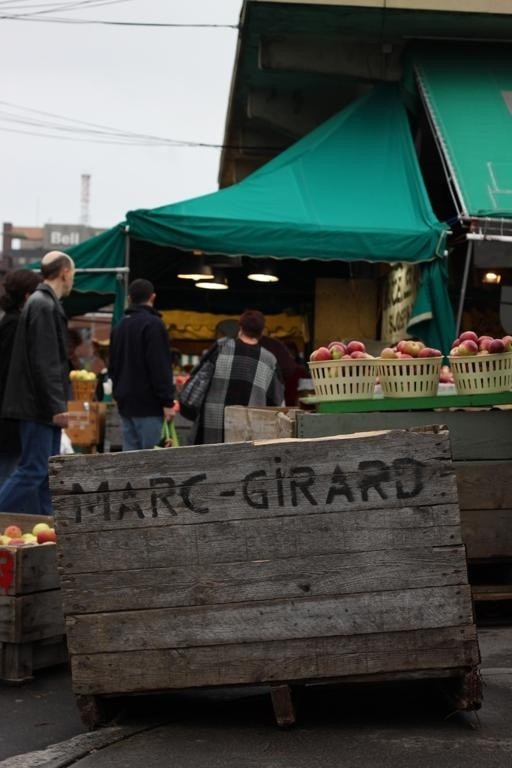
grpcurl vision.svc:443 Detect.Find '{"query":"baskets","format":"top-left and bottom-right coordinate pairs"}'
top-left (447, 349), bottom-right (512, 393)
top-left (377, 353), bottom-right (443, 398)
top-left (308, 358), bottom-right (377, 400)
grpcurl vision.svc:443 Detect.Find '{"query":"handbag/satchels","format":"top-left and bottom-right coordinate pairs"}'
top-left (180, 339), bottom-right (228, 421)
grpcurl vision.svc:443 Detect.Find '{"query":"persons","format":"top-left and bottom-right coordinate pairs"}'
top-left (180, 310), bottom-right (285, 448)
top-left (1, 269), bottom-right (39, 476)
top-left (213, 314), bottom-right (303, 407)
top-left (109, 277), bottom-right (185, 452)
top-left (2, 250), bottom-right (76, 513)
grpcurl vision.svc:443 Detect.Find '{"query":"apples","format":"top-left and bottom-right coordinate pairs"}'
top-left (480, 340), bottom-right (481, 341)
top-left (381, 341), bottom-right (441, 359)
top-left (439, 366), bottom-right (455, 384)
top-left (311, 341), bottom-right (367, 361)
top-left (450, 331), bottom-right (512, 356)
top-left (0, 523), bottom-right (56, 546)
